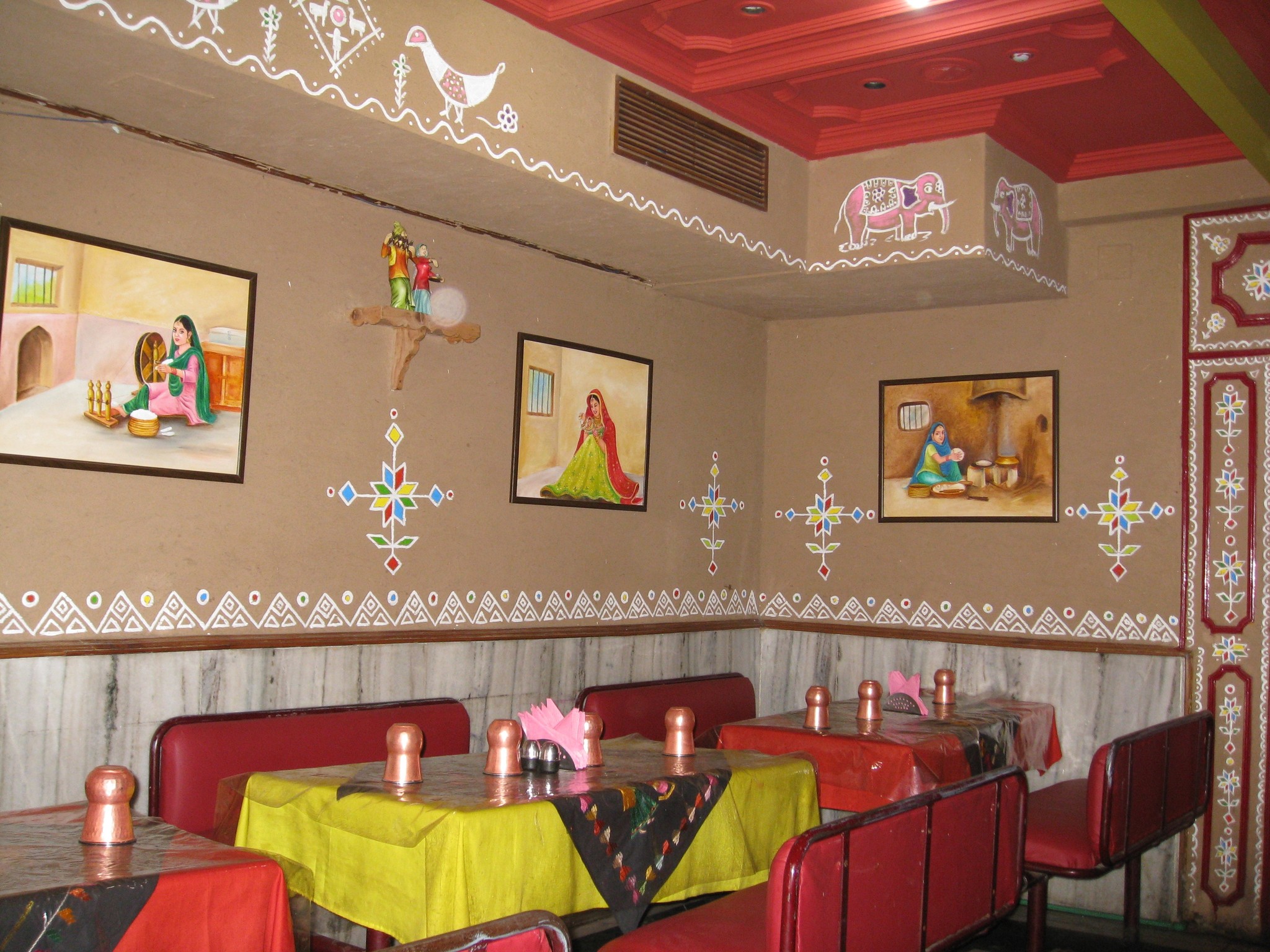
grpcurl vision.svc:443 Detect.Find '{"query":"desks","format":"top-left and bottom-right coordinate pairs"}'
top-left (721, 687), bottom-right (1062, 816)
top-left (0, 800), bottom-right (296, 952)
top-left (233, 735), bottom-right (820, 952)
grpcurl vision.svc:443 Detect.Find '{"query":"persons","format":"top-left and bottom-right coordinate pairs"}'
top-left (381, 221), bottom-right (444, 314)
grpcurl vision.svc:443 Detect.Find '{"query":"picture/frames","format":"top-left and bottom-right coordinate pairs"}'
top-left (0, 215), bottom-right (258, 484)
top-left (509, 331), bottom-right (654, 513)
top-left (877, 369), bottom-right (1061, 524)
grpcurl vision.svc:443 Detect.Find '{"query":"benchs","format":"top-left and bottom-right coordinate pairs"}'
top-left (597, 764), bottom-right (1029, 952)
top-left (576, 672), bottom-right (757, 742)
top-left (148, 697), bottom-right (471, 846)
top-left (1023, 710), bottom-right (1216, 952)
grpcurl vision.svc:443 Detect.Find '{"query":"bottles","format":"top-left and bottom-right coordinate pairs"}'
top-left (539, 741), bottom-right (561, 773)
top-left (520, 739), bottom-right (540, 771)
